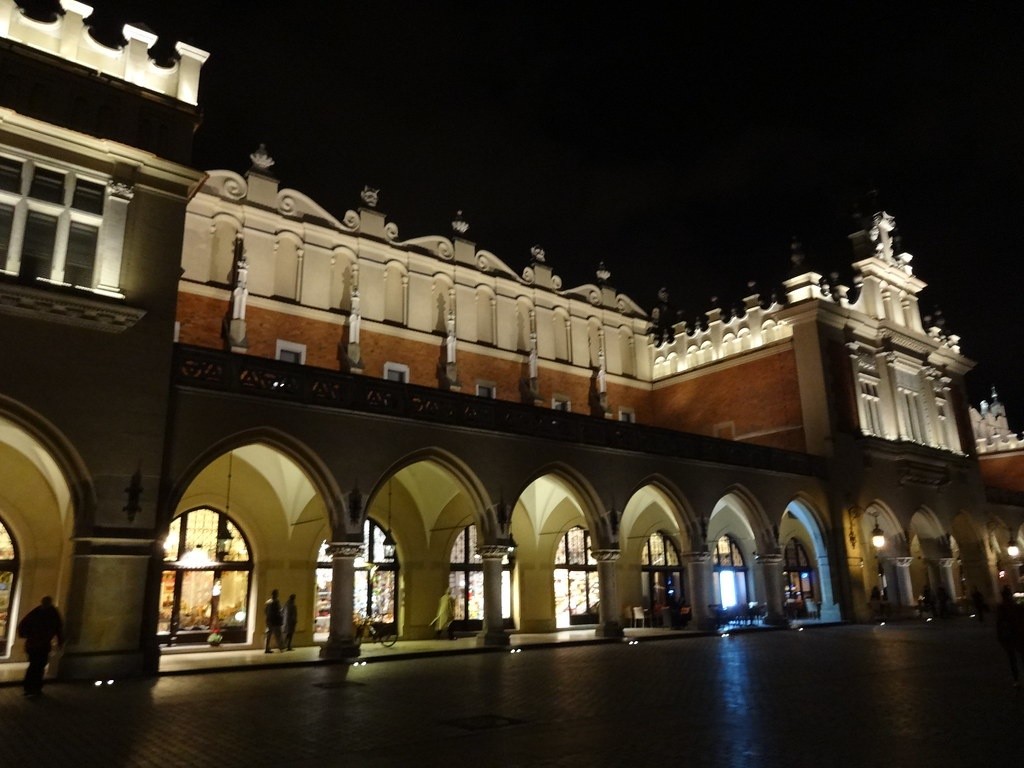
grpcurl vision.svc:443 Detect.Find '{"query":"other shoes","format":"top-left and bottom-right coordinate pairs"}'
top-left (1012, 683), bottom-right (1022, 688)
top-left (265, 650), bottom-right (273, 653)
top-left (25, 692), bottom-right (46, 704)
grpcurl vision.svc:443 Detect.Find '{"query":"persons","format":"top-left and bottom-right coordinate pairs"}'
top-left (264, 589), bottom-right (286, 653)
top-left (17, 596), bottom-right (66, 698)
top-left (284, 593), bottom-right (297, 651)
top-left (434, 588), bottom-right (456, 640)
top-left (871, 586), bottom-right (881, 616)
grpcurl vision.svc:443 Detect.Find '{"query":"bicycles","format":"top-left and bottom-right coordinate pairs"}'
top-left (355, 610), bottom-right (399, 648)
